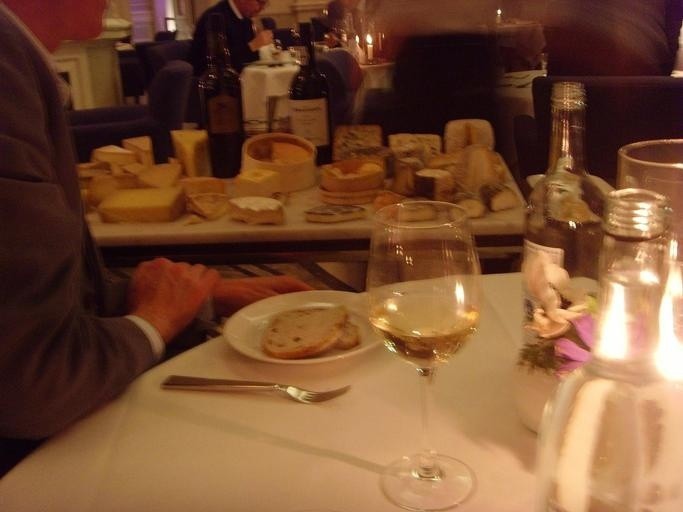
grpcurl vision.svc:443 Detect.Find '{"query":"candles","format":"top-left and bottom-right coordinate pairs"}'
top-left (365, 34), bottom-right (373, 59)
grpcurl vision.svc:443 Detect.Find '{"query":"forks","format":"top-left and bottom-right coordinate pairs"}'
top-left (157, 373), bottom-right (353, 406)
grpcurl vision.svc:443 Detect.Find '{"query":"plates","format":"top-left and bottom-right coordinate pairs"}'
top-left (217, 287), bottom-right (383, 368)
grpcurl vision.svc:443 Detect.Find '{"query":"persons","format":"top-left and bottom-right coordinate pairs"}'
top-left (538, 1), bottom-right (683, 78)
top-left (139, 0), bottom-right (339, 175)
top-left (1, 1), bottom-right (318, 475)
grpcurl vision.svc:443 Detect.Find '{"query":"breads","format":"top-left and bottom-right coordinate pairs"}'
top-left (305, 119), bottom-right (519, 224)
top-left (69, 118), bottom-right (316, 226)
top-left (261, 303), bottom-right (360, 360)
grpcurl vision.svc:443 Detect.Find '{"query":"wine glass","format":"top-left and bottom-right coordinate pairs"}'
top-left (363, 197), bottom-right (482, 512)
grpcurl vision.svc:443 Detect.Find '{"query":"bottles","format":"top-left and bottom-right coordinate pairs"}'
top-left (195, 12), bottom-right (242, 180)
top-left (287, 35), bottom-right (333, 167)
top-left (520, 75), bottom-right (606, 365)
top-left (529, 184), bottom-right (683, 512)
